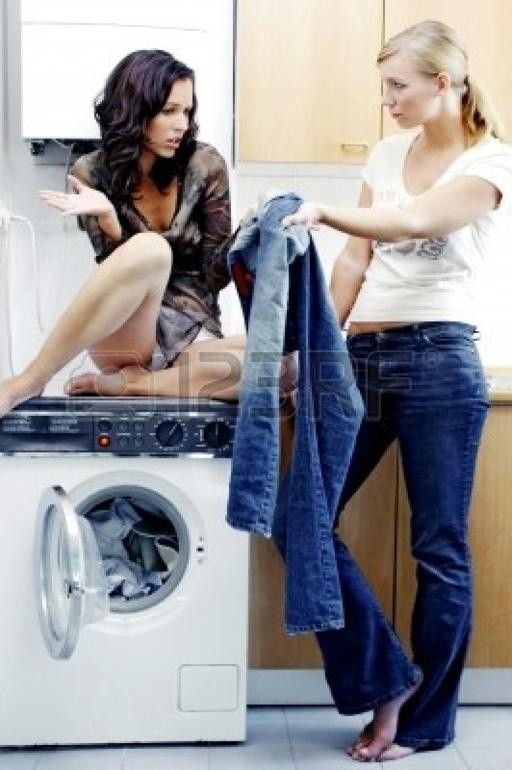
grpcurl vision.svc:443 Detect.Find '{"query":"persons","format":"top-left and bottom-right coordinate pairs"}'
top-left (1, 49), bottom-right (299, 420)
top-left (271, 21), bottom-right (509, 763)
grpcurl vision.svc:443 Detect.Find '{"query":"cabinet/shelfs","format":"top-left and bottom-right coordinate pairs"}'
top-left (246, 393), bottom-right (511, 705)
top-left (234, 0), bottom-right (511, 164)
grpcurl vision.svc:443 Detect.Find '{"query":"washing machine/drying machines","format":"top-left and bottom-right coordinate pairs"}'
top-left (0, 395), bottom-right (252, 748)
